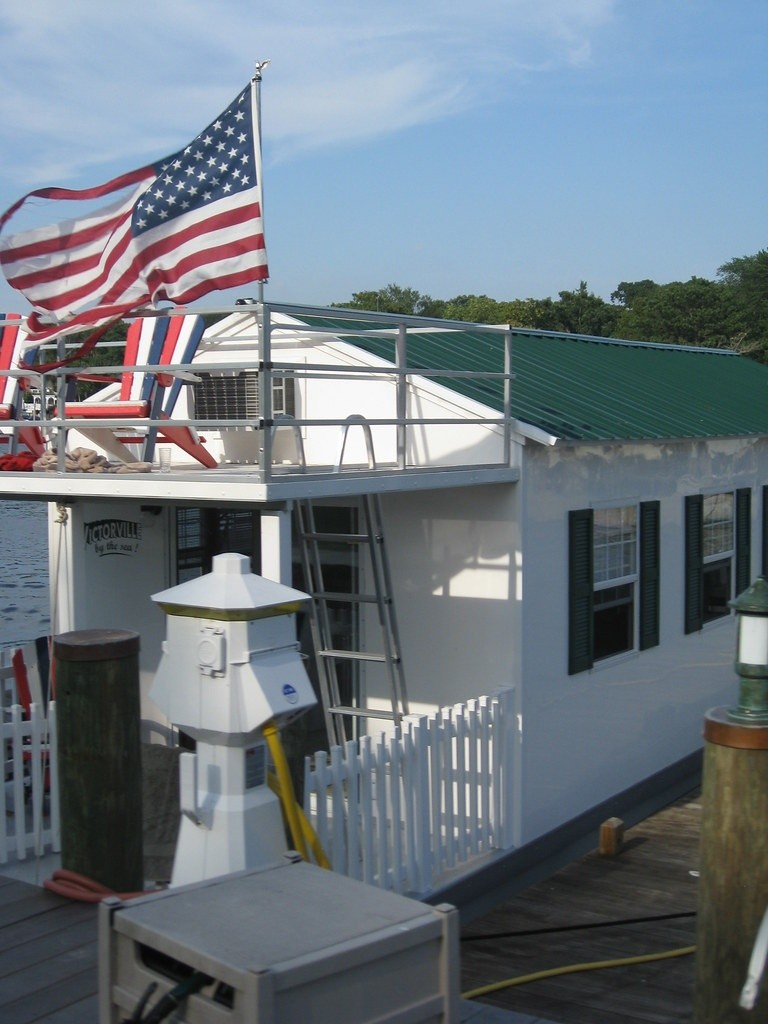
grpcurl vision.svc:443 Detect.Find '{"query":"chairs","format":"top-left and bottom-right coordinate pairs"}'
top-left (0, 306), bottom-right (219, 469)
top-left (13, 636), bottom-right (53, 818)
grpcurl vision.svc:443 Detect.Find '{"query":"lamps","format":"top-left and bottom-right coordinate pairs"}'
top-left (728, 573), bottom-right (767, 725)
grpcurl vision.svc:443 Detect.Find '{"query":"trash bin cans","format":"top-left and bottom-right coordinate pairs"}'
top-left (95, 850), bottom-right (460, 1023)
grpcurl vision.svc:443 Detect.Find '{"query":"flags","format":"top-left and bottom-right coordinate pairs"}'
top-left (0, 79), bottom-right (270, 327)
